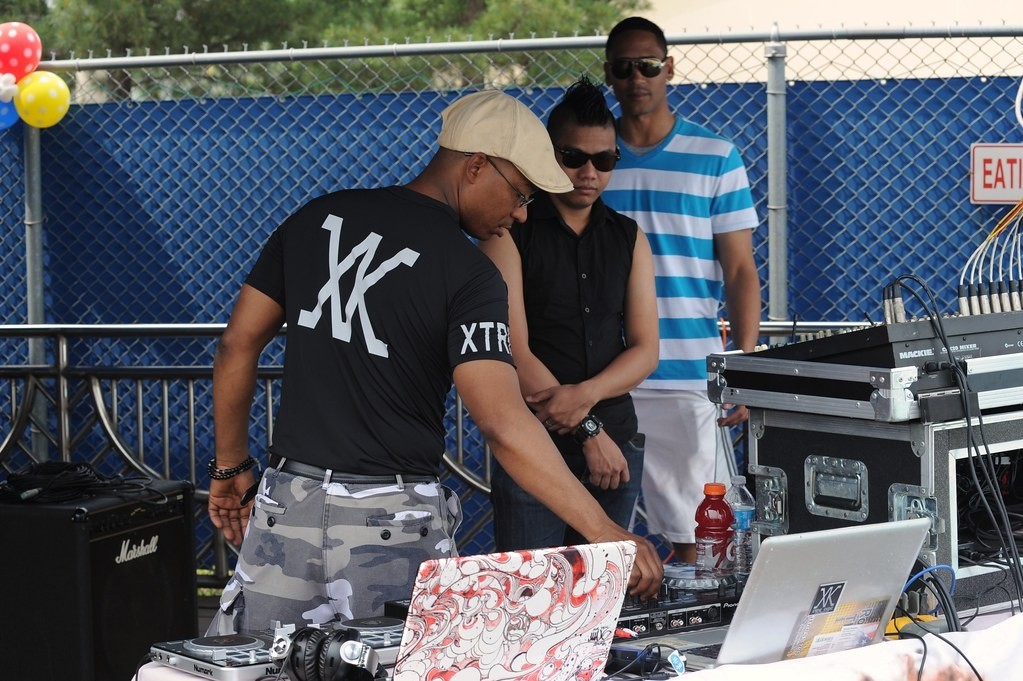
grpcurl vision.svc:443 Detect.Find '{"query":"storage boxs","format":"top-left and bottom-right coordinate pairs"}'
top-left (700, 350), bottom-right (1023, 426)
top-left (743, 409), bottom-right (1023, 617)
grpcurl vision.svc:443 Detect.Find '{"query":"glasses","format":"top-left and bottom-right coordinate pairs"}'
top-left (606, 56), bottom-right (668, 79)
top-left (555, 144), bottom-right (621, 173)
top-left (463, 153), bottom-right (534, 208)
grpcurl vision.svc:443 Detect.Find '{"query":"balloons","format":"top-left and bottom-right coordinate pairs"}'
top-left (0, 22), bottom-right (71, 131)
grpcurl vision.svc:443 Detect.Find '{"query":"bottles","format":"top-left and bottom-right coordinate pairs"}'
top-left (727, 476), bottom-right (756, 579)
top-left (696, 482), bottom-right (735, 571)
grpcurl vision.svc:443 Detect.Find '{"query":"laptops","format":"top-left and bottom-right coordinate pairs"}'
top-left (617, 519), bottom-right (933, 673)
top-left (391, 541), bottom-right (637, 681)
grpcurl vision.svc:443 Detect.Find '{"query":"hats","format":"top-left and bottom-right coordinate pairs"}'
top-left (437, 90), bottom-right (575, 193)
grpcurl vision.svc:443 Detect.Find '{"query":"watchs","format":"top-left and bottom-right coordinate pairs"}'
top-left (571, 415), bottom-right (604, 451)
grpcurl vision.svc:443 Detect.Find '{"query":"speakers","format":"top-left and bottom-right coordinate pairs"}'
top-left (0, 479), bottom-right (198, 681)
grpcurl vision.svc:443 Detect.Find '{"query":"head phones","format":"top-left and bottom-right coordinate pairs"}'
top-left (272, 627), bottom-right (389, 681)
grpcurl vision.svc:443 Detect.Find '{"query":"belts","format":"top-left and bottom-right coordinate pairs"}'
top-left (239, 456), bottom-right (437, 506)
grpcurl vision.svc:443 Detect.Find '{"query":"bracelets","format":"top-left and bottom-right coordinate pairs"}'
top-left (206, 456), bottom-right (256, 480)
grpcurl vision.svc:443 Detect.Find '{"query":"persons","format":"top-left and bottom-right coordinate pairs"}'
top-left (203, 89), bottom-right (666, 637)
top-left (478, 76), bottom-right (661, 550)
top-left (598, 15), bottom-right (761, 572)
